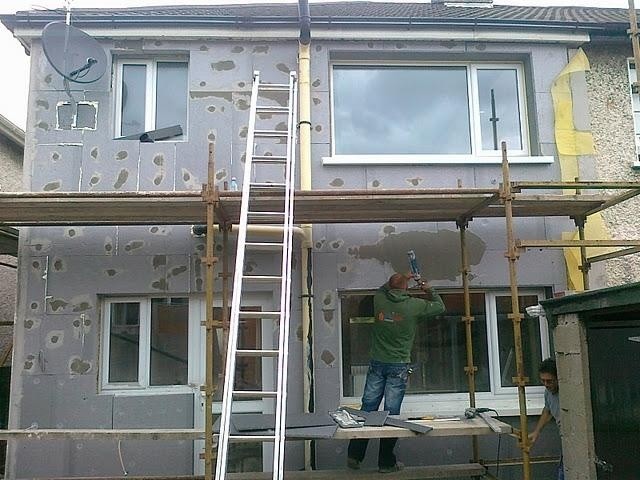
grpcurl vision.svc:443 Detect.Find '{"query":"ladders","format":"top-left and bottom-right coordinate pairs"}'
top-left (216, 71), bottom-right (297, 480)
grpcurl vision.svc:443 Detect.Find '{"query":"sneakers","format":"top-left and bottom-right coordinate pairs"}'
top-left (379, 462), bottom-right (404, 472)
top-left (348, 456), bottom-right (360, 470)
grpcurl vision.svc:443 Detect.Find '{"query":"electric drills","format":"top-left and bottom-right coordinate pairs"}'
top-left (464, 408), bottom-right (490, 420)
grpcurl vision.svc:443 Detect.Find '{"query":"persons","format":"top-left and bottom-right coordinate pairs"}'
top-left (347, 274), bottom-right (446, 472)
top-left (528, 357), bottom-right (564, 480)
